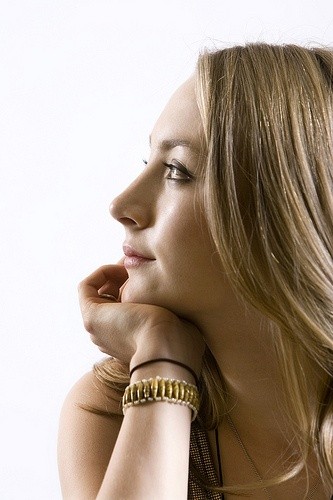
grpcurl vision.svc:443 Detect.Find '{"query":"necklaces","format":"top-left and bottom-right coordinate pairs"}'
top-left (221, 403), bottom-right (324, 500)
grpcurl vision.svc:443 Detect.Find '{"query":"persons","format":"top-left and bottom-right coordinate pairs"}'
top-left (54, 42), bottom-right (333, 499)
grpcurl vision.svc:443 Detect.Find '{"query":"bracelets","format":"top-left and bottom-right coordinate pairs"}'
top-left (128, 358), bottom-right (202, 391)
top-left (120, 377), bottom-right (200, 423)
